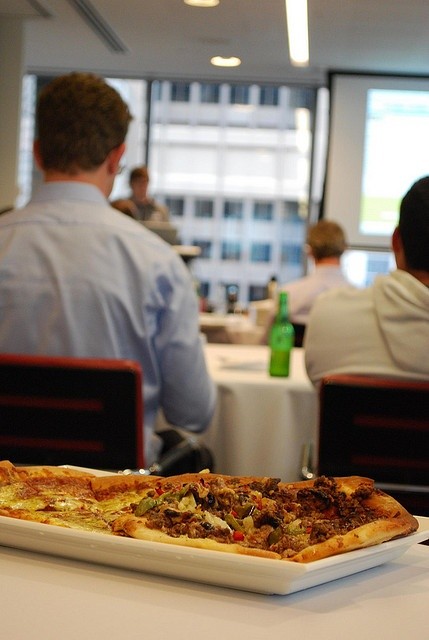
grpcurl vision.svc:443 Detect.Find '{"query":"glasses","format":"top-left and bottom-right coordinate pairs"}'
top-left (115, 159), bottom-right (124, 175)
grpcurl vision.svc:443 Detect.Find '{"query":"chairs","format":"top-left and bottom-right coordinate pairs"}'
top-left (293, 321), bottom-right (307, 347)
top-left (0, 352), bottom-right (213, 475)
top-left (300, 374), bottom-right (429, 499)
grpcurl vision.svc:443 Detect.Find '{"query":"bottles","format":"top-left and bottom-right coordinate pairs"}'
top-left (269, 291), bottom-right (293, 382)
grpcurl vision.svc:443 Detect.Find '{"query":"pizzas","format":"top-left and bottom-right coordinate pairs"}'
top-left (0, 458), bottom-right (419, 564)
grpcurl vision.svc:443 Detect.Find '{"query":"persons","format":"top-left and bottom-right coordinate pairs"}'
top-left (301, 173), bottom-right (428, 392)
top-left (0, 68), bottom-right (219, 474)
top-left (109, 167), bottom-right (170, 225)
top-left (274, 219), bottom-right (359, 327)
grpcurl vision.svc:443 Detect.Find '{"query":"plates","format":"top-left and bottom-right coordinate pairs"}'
top-left (1, 466), bottom-right (429, 597)
